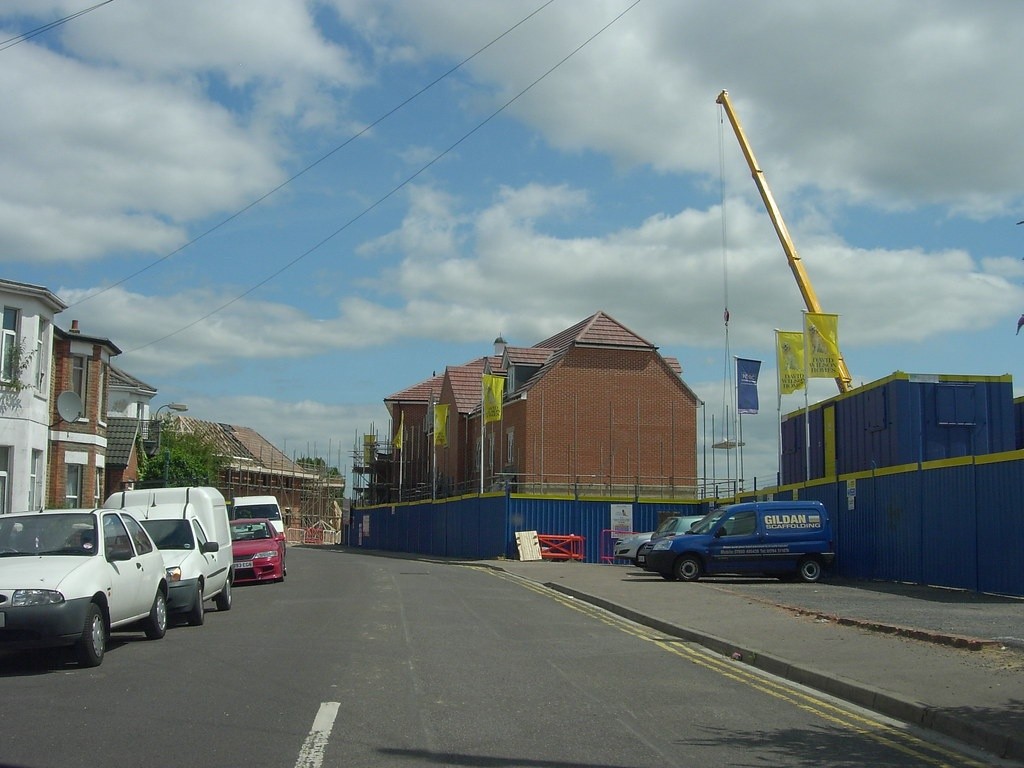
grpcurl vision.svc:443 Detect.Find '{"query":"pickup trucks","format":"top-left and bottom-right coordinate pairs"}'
top-left (98, 485), bottom-right (235, 626)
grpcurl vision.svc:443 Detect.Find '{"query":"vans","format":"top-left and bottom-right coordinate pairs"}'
top-left (231, 495), bottom-right (290, 556)
top-left (648, 514), bottom-right (735, 540)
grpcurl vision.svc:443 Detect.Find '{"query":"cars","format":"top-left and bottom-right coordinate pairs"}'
top-left (613, 531), bottom-right (651, 567)
top-left (1, 508), bottom-right (168, 668)
top-left (225, 517), bottom-right (286, 587)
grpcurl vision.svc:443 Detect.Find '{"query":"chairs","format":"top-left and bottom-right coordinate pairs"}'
top-left (254, 529), bottom-right (266, 538)
top-left (237, 509), bottom-right (252, 518)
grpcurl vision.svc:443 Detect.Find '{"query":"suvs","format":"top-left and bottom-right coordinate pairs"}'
top-left (637, 499), bottom-right (832, 583)
